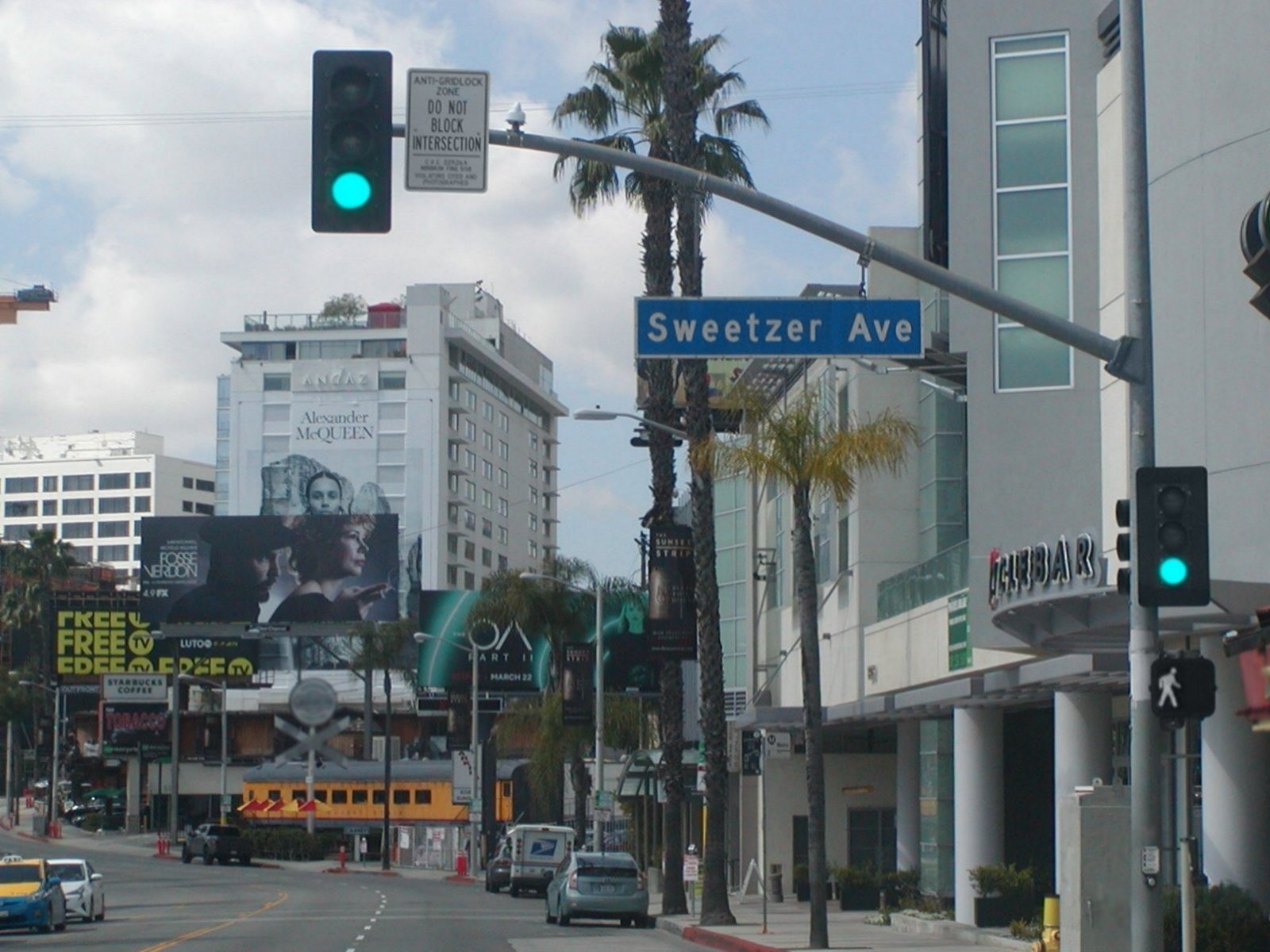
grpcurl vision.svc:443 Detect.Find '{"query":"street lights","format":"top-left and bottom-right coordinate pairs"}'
top-left (177, 673), bottom-right (227, 825)
top-left (518, 569), bottom-right (604, 852)
top-left (18, 680), bottom-right (59, 839)
top-left (414, 632), bottom-right (479, 878)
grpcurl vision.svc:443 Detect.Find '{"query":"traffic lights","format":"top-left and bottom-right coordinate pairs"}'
top-left (312, 48), bottom-right (394, 235)
top-left (1151, 656), bottom-right (1212, 721)
top-left (1115, 498), bottom-right (1131, 599)
top-left (1138, 467), bottom-right (1212, 609)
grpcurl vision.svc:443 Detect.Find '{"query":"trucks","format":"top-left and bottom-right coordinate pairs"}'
top-left (506, 823), bottom-right (577, 899)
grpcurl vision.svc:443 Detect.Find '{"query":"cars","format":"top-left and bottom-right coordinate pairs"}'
top-left (45, 858), bottom-right (106, 923)
top-left (63, 794), bottom-right (144, 832)
top-left (0, 853), bottom-right (67, 935)
top-left (544, 851), bottom-right (649, 929)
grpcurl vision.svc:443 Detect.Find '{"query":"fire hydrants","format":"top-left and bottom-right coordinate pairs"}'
top-left (1031, 891), bottom-right (1061, 952)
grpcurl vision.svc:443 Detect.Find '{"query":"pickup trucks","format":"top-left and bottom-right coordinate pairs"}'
top-left (181, 824), bottom-right (251, 865)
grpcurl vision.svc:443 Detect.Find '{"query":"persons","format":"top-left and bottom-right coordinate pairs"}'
top-left (161, 472), bottom-right (395, 625)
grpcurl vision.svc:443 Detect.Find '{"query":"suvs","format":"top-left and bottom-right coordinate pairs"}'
top-left (485, 841), bottom-right (513, 894)
